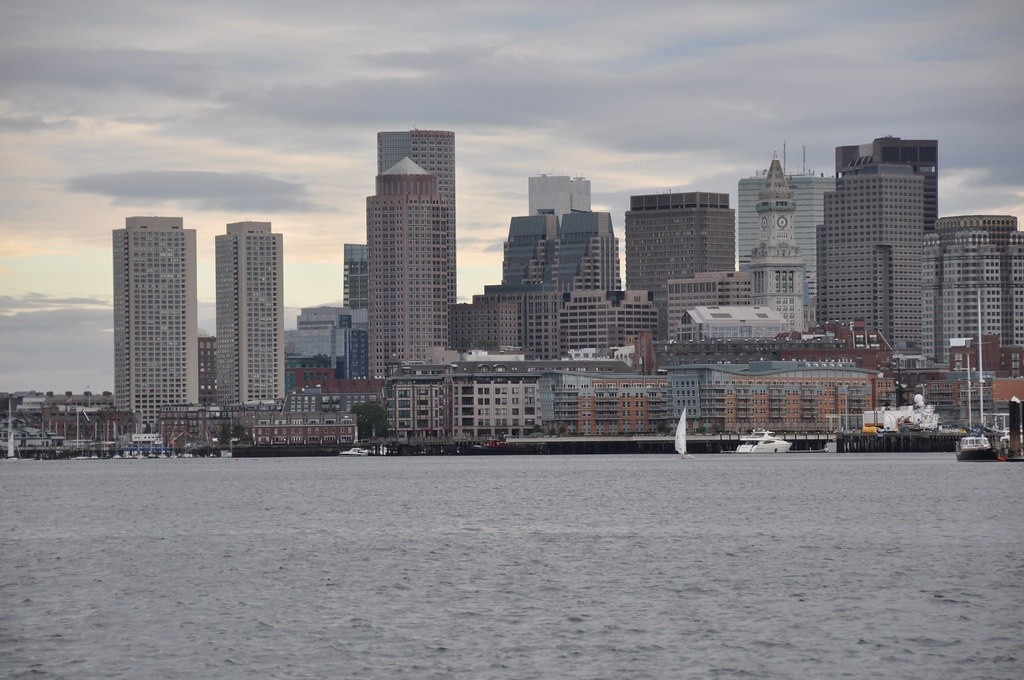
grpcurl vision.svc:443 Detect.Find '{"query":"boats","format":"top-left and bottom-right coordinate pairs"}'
top-left (734, 429), bottom-right (794, 454)
top-left (954, 291), bottom-right (1024, 461)
top-left (339, 446), bottom-right (368, 456)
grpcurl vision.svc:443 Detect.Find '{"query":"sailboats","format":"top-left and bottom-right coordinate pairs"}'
top-left (6, 409), bottom-right (194, 461)
top-left (674, 407), bottom-right (696, 459)
